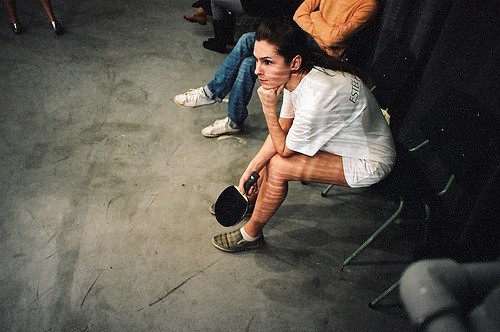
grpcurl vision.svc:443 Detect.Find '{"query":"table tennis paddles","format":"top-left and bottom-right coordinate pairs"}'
top-left (214, 170), bottom-right (261, 227)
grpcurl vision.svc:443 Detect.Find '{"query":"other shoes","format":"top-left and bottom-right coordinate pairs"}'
top-left (203, 38), bottom-right (227, 54)
top-left (183, 7), bottom-right (208, 25)
top-left (211, 228), bottom-right (265, 252)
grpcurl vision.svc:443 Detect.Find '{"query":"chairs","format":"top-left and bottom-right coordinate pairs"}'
top-left (321, 85), bottom-right (489, 271)
top-left (282, 38), bottom-right (420, 185)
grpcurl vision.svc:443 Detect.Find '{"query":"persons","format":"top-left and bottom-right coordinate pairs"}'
top-left (209, 18), bottom-right (396, 252)
top-left (174, 0), bottom-right (380, 137)
top-left (3, 0), bottom-right (64, 32)
top-left (183, 0), bottom-right (304, 53)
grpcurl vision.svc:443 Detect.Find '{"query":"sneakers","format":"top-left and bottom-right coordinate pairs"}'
top-left (202, 117), bottom-right (240, 137)
top-left (174, 86), bottom-right (216, 108)
